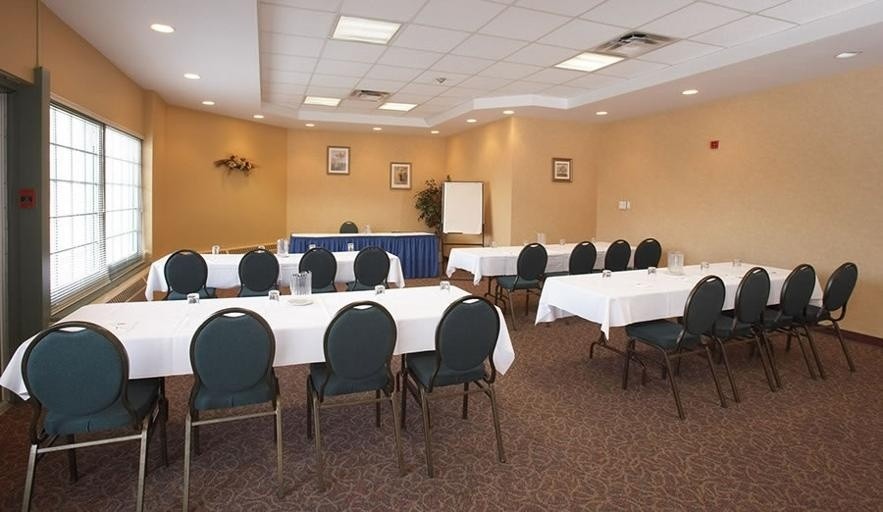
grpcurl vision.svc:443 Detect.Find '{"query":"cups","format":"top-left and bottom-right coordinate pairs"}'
top-left (187, 271), bottom-right (451, 306)
top-left (491, 232), bottom-right (596, 248)
top-left (601, 251), bottom-right (741, 281)
top-left (211, 239), bottom-right (354, 257)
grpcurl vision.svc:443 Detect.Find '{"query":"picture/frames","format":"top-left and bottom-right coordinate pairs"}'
top-left (326, 146), bottom-right (350, 174)
top-left (390, 162), bottom-right (412, 189)
top-left (552, 157), bottom-right (573, 182)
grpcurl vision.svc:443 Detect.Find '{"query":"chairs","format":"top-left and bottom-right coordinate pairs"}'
top-left (495, 238), bottom-right (662, 331)
top-left (21, 321), bottom-right (169, 512)
top-left (622, 262), bottom-right (858, 420)
top-left (183, 307), bottom-right (285, 512)
top-left (400, 296), bottom-right (507, 478)
top-left (339, 222), bottom-right (358, 233)
top-left (163, 247), bottom-right (390, 300)
top-left (302, 302), bottom-right (407, 493)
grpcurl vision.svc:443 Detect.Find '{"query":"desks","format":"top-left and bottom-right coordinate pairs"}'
top-left (534, 262), bottom-right (824, 386)
top-left (445, 242), bottom-right (636, 314)
top-left (290, 232), bottom-right (438, 278)
top-left (145, 252), bottom-right (404, 301)
top-left (0, 286), bottom-right (516, 449)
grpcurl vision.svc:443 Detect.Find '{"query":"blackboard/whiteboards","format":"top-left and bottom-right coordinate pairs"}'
top-left (441, 181), bottom-right (484, 235)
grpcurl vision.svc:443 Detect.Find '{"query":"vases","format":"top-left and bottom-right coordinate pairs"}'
top-left (410, 174), bottom-right (451, 262)
